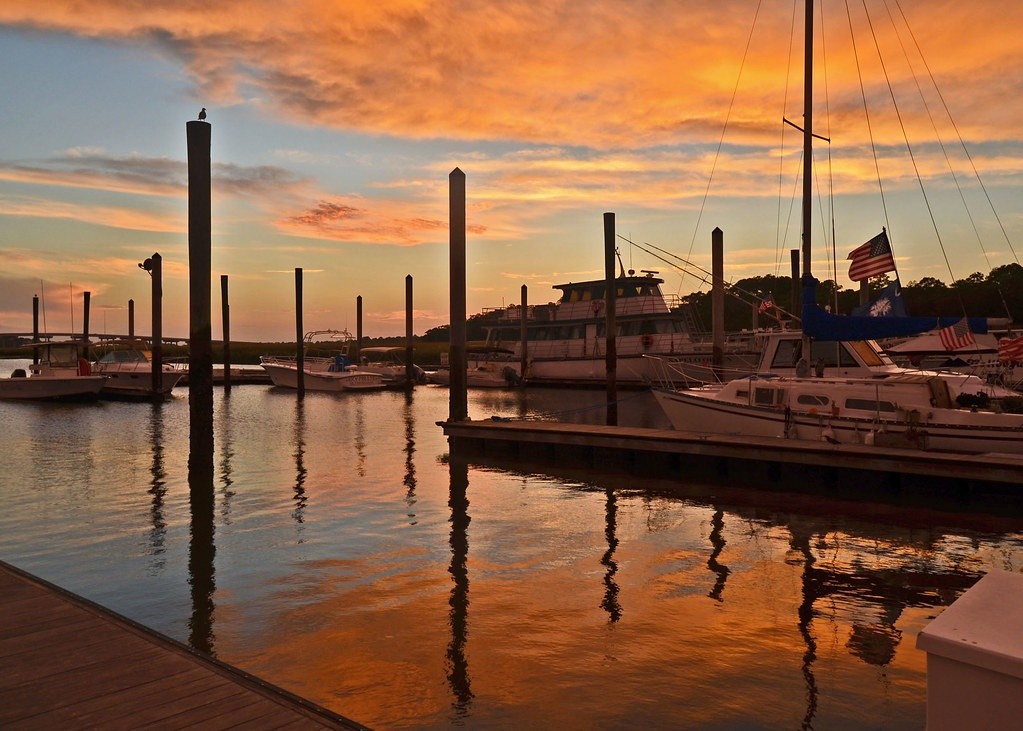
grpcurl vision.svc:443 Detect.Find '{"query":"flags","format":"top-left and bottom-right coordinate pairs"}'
top-left (848, 278), bottom-right (911, 317)
top-left (846, 230), bottom-right (895, 282)
top-left (757, 293), bottom-right (776, 314)
top-left (938, 315), bottom-right (975, 351)
top-left (998, 333), bottom-right (1023, 361)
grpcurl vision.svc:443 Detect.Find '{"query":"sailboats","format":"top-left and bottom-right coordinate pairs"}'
top-left (636, 0), bottom-right (1023, 446)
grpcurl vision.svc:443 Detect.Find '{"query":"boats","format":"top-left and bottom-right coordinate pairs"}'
top-left (343, 346), bottom-right (429, 385)
top-left (475, 245), bottom-right (763, 391)
top-left (0, 343), bottom-right (110, 399)
top-left (429, 345), bottom-right (523, 387)
top-left (88, 346), bottom-right (186, 397)
top-left (259, 330), bottom-right (392, 392)
